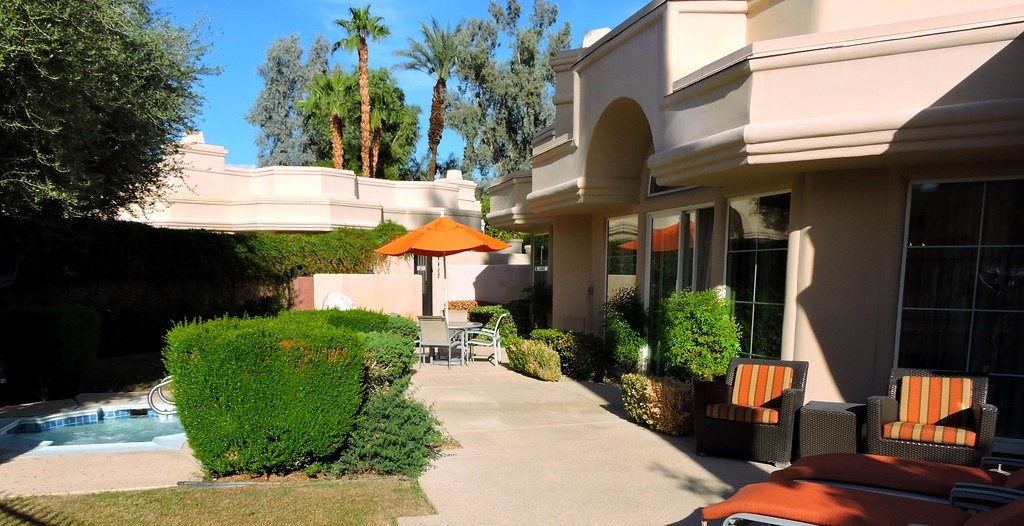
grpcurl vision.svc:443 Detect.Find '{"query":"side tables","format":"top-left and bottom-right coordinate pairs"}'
top-left (799, 398), bottom-right (867, 458)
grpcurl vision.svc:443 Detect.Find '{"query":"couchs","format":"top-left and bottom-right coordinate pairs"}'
top-left (866, 368), bottom-right (998, 470)
top-left (694, 358), bottom-right (809, 463)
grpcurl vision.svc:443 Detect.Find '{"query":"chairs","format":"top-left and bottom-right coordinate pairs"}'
top-left (418, 315), bottom-right (465, 368)
top-left (440, 309), bottom-right (472, 361)
top-left (386, 312), bottom-right (436, 364)
top-left (466, 313), bottom-right (508, 366)
top-left (771, 452), bottom-right (1024, 516)
top-left (700, 482), bottom-right (1023, 526)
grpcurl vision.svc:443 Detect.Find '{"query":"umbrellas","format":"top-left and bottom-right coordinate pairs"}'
top-left (374, 211), bottom-right (512, 322)
top-left (616, 220), bottom-right (696, 258)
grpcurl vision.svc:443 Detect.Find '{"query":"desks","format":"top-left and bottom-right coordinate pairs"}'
top-left (430, 322), bottom-right (483, 364)
